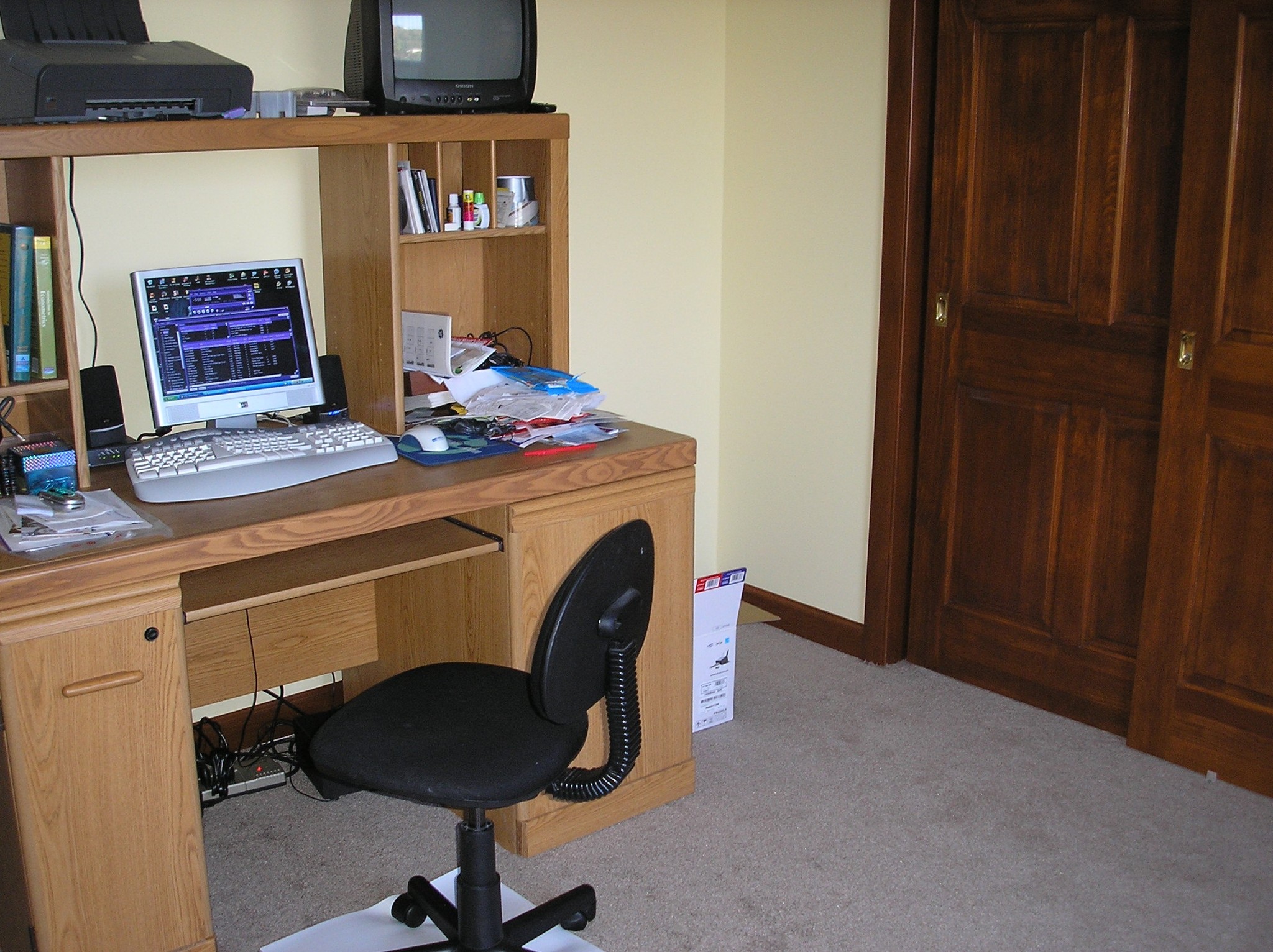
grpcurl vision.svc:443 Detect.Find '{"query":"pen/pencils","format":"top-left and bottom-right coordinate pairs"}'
top-left (523, 443), bottom-right (598, 457)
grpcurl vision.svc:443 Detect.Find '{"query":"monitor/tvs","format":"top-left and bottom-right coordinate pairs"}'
top-left (343, 0), bottom-right (537, 116)
top-left (130, 258), bottom-right (325, 428)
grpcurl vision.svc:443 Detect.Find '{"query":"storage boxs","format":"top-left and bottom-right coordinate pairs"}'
top-left (692, 567), bottom-right (782, 734)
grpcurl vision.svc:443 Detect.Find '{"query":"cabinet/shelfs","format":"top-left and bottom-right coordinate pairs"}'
top-left (0, 108), bottom-right (573, 498)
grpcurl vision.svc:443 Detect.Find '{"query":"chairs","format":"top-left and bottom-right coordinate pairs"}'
top-left (308, 516), bottom-right (655, 952)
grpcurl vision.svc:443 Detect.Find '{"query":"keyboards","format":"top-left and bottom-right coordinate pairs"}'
top-left (123, 421), bottom-right (399, 503)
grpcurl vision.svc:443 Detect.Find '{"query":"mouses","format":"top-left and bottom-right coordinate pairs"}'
top-left (398, 424), bottom-right (449, 452)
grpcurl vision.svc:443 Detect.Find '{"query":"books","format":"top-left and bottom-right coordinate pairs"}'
top-left (0, 223), bottom-right (57, 386)
top-left (403, 390), bottom-right (456, 412)
top-left (397, 160), bottom-right (441, 234)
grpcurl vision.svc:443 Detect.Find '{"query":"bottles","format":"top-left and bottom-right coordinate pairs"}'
top-left (473, 193), bottom-right (490, 229)
top-left (447, 193), bottom-right (461, 230)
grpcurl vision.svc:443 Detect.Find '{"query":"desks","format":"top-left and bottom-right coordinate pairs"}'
top-left (0, 391), bottom-right (698, 952)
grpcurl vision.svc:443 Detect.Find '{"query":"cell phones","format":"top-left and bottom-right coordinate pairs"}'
top-left (38, 486), bottom-right (86, 512)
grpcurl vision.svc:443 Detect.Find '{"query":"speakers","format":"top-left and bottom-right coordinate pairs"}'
top-left (78, 365), bottom-right (126, 448)
top-left (308, 354), bottom-right (349, 424)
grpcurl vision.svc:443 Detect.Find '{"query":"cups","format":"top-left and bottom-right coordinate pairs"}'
top-left (496, 176), bottom-right (539, 226)
